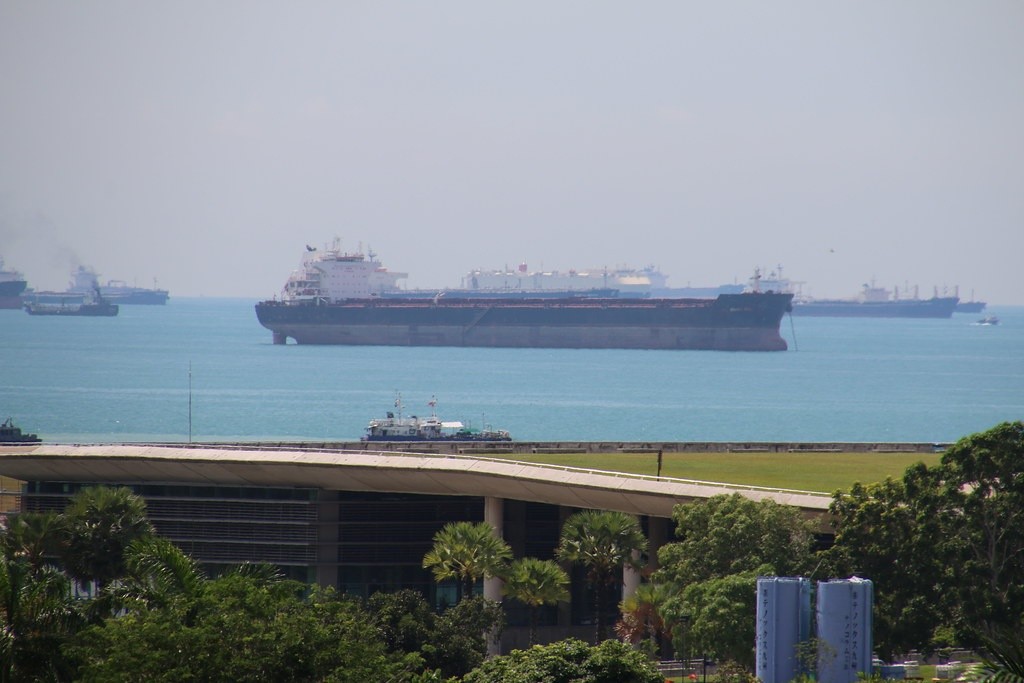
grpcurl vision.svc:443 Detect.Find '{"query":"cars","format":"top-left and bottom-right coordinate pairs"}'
top-left (527, 575), bottom-right (622, 626)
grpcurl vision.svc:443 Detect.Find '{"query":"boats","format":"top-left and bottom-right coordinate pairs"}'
top-left (956, 290), bottom-right (986, 314)
top-left (24, 266), bottom-right (169, 306)
top-left (790, 286), bottom-right (960, 319)
top-left (359, 394), bottom-right (512, 441)
top-left (23, 288), bottom-right (118, 317)
top-left (0, 417), bottom-right (42, 446)
top-left (0, 256), bottom-right (27, 296)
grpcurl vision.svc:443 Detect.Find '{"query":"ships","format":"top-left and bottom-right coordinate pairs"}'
top-left (255, 245), bottom-right (795, 352)
